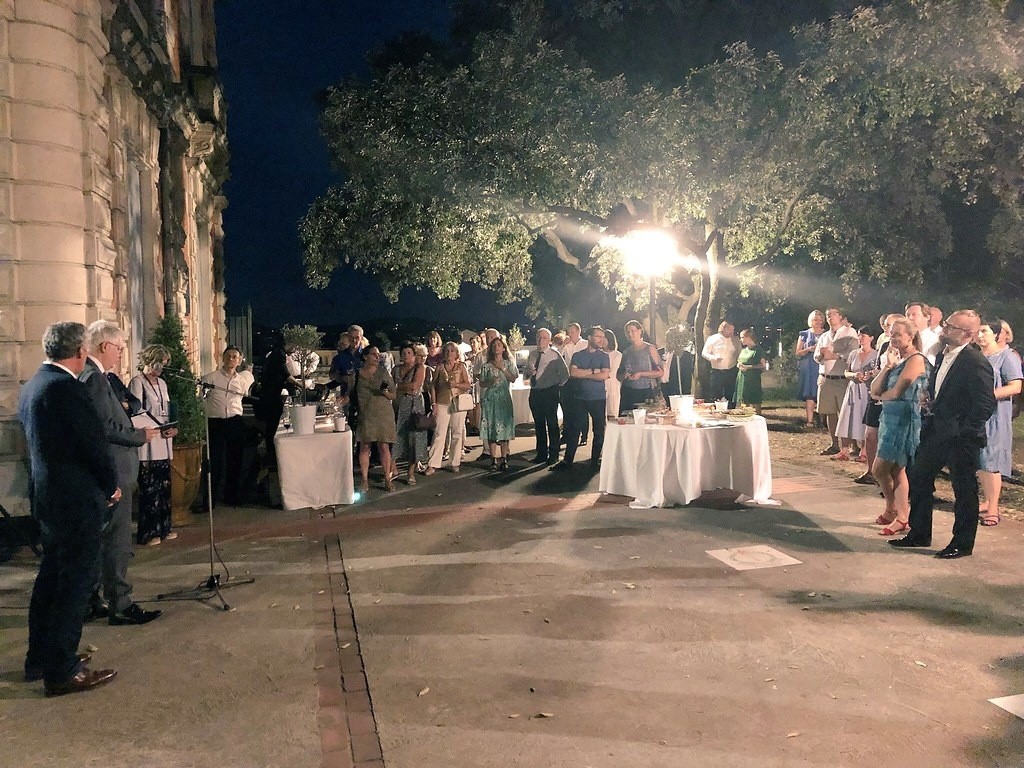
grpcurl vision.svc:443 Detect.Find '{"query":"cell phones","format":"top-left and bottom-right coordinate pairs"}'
top-left (380, 382), bottom-right (388, 390)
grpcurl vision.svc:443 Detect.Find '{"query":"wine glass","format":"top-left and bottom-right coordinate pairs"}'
top-left (625, 364), bottom-right (632, 381)
top-left (918, 387), bottom-right (935, 417)
top-left (869, 359), bottom-right (879, 371)
top-left (282, 420), bottom-right (291, 437)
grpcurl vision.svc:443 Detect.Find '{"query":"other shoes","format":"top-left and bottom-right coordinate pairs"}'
top-left (147, 529), bottom-right (177, 545)
top-left (128, 552), bottom-right (134, 558)
top-left (424, 467), bottom-right (459, 475)
top-left (801, 421), bottom-right (881, 484)
top-left (192, 506), bottom-right (215, 513)
top-left (389, 473), bottom-right (398, 481)
top-left (530, 439), bottom-right (601, 471)
top-left (476, 453), bottom-right (509, 472)
top-left (408, 477), bottom-right (416, 486)
top-left (385, 482), bottom-right (396, 492)
top-left (358, 480), bottom-right (369, 493)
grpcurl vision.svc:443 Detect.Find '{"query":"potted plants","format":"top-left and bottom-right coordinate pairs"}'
top-left (147, 314), bottom-right (208, 528)
top-left (328, 397), bottom-right (351, 431)
top-left (665, 314), bottom-right (696, 414)
top-left (715, 397), bottom-right (730, 412)
top-left (281, 324), bottom-right (326, 437)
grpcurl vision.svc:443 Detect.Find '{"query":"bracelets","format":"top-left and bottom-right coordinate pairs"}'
top-left (592, 368), bottom-right (594, 374)
top-left (503, 368), bottom-right (506, 371)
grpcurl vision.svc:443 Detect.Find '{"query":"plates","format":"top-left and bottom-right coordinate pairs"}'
top-left (655, 409), bottom-right (760, 429)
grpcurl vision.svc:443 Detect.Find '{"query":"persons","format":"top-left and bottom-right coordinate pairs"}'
top-left (732, 328), bottom-right (766, 415)
top-left (314, 325), bottom-right (519, 494)
top-left (649, 328), bottom-right (694, 407)
top-left (127, 344), bottom-right (178, 546)
top-left (601, 329), bottom-right (622, 420)
top-left (886, 310), bottom-right (996, 559)
top-left (84, 319), bottom-right (162, 625)
top-left (286, 342), bottom-right (319, 379)
top-left (701, 320), bottom-right (742, 409)
top-left (796, 302), bottom-right (1023, 536)
top-left (188, 346), bottom-right (248, 514)
top-left (972, 313), bottom-right (1024, 526)
top-left (250, 332), bottom-right (307, 473)
top-left (16, 321), bottom-right (122, 697)
top-left (549, 327), bottom-right (611, 472)
top-left (616, 320), bottom-right (664, 418)
top-left (522, 328), bottom-right (553, 465)
top-left (557, 323), bottom-right (589, 445)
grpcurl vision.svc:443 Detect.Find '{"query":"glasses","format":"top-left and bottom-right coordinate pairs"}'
top-left (107, 341), bottom-right (124, 354)
top-left (942, 320), bottom-right (966, 331)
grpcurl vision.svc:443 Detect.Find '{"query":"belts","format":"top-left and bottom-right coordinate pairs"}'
top-left (818, 373), bottom-right (846, 379)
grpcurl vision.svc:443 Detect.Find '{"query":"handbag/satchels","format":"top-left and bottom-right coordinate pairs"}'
top-left (653, 390), bottom-right (666, 405)
top-left (409, 412), bottom-right (437, 431)
top-left (453, 393), bottom-right (473, 411)
top-left (348, 389), bottom-right (360, 430)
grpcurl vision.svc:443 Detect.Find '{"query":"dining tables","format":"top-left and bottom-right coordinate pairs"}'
top-left (512, 375), bottom-right (564, 428)
top-left (599, 413), bottom-right (773, 506)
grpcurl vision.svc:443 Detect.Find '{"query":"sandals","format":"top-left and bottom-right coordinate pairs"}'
top-left (979, 503), bottom-right (1001, 526)
top-left (876, 510), bottom-right (910, 535)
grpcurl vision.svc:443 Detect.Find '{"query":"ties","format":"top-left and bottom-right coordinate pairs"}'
top-left (530, 352), bottom-right (543, 387)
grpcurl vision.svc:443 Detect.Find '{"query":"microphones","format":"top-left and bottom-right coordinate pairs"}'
top-left (153, 363), bottom-right (185, 373)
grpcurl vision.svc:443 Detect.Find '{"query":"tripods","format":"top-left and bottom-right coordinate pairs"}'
top-left (158, 371), bottom-right (260, 609)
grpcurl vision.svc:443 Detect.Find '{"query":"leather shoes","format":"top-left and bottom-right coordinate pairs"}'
top-left (887, 535), bottom-right (972, 559)
top-left (109, 604), bottom-right (162, 626)
top-left (85, 599), bottom-right (109, 622)
top-left (44, 666), bottom-right (117, 697)
top-left (24, 653), bottom-right (91, 682)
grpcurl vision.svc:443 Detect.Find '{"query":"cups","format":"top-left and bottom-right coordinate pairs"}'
top-left (633, 408), bottom-right (646, 426)
top-left (324, 393), bottom-right (346, 432)
top-left (715, 401), bottom-right (729, 410)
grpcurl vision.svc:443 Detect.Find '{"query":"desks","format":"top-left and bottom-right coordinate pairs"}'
top-left (274, 396), bottom-right (353, 510)
